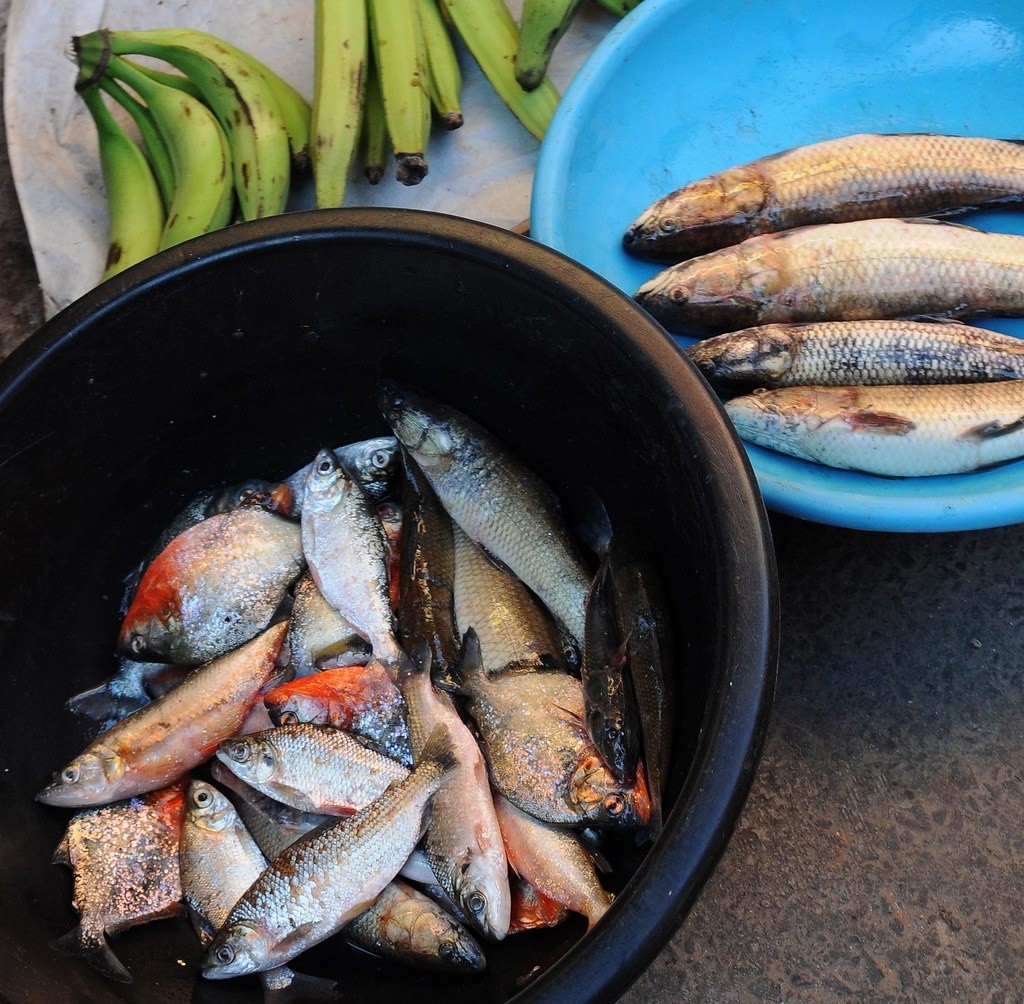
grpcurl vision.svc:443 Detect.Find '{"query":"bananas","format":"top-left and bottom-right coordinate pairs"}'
top-left (72, 0), bottom-right (645, 282)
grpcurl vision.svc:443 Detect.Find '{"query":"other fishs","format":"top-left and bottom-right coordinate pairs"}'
top-left (32, 379), bottom-right (672, 1004)
top-left (620, 127), bottom-right (1024, 479)
